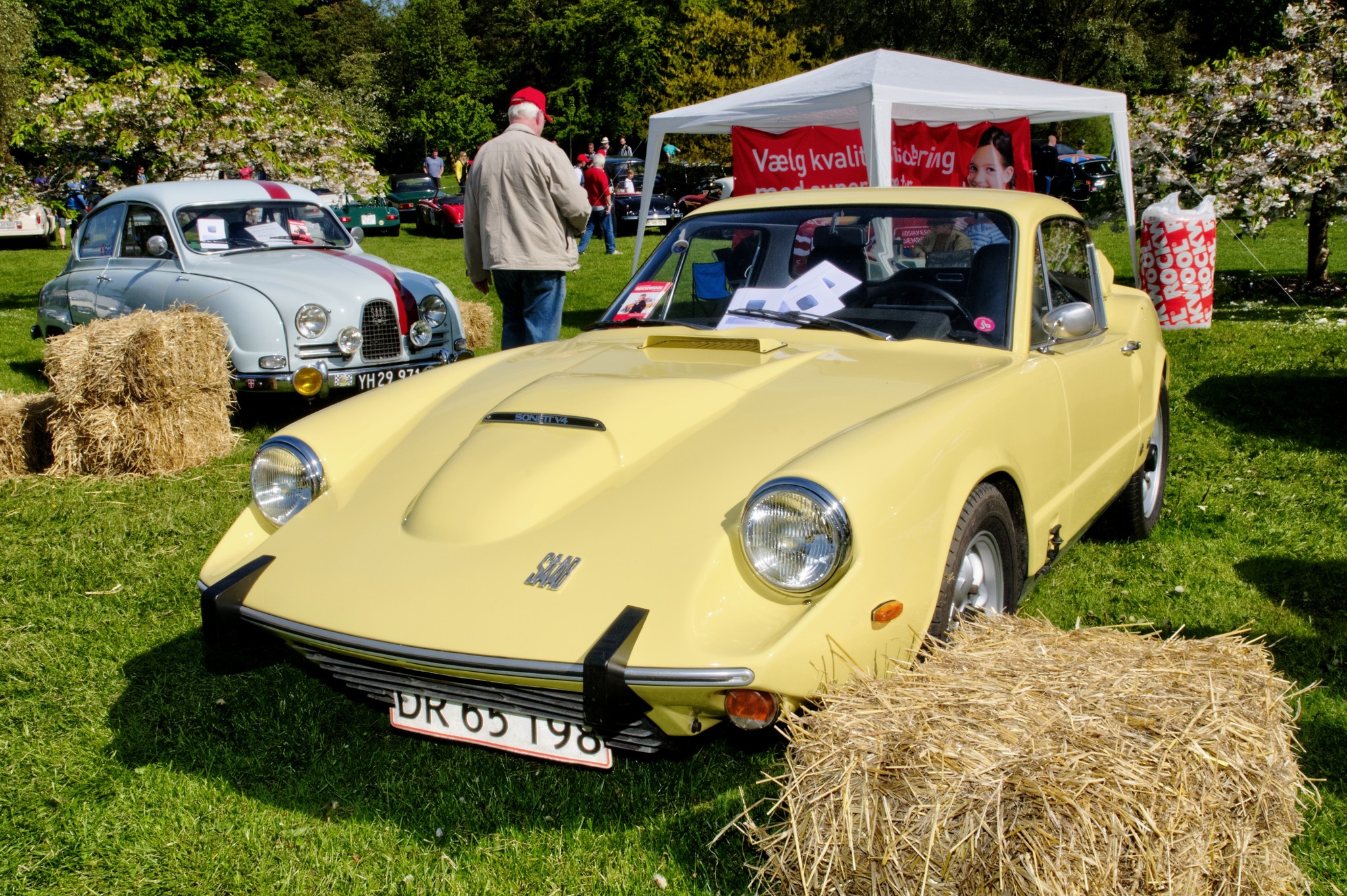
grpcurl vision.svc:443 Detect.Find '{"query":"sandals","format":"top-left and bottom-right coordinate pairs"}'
top-left (61, 245), bottom-right (67, 250)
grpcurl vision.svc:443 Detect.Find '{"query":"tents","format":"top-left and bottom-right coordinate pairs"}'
top-left (629, 47), bottom-right (1140, 286)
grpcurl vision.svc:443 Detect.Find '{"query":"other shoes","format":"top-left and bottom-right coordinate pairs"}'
top-left (578, 251), bottom-right (585, 255)
top-left (611, 250), bottom-right (623, 254)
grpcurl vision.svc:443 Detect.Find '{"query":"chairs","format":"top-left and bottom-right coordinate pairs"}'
top-left (711, 247), bottom-right (747, 294)
top-left (691, 260), bottom-right (734, 317)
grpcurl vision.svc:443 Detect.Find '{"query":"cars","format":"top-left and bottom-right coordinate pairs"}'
top-left (28, 176), bottom-right (475, 418)
top-left (603, 155), bottom-right (683, 236)
top-left (1028, 138), bottom-right (1115, 211)
top-left (676, 175), bottom-right (735, 214)
top-left (0, 187), bottom-right (58, 247)
top-left (309, 182), bottom-right (355, 212)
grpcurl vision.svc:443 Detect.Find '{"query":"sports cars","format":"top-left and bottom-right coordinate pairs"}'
top-left (328, 192), bottom-right (402, 238)
top-left (415, 186), bottom-right (466, 234)
top-left (374, 173), bottom-right (446, 222)
top-left (194, 184), bottom-right (1174, 773)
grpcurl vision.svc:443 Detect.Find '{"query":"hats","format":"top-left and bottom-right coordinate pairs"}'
top-left (600, 137), bottom-right (610, 143)
top-left (577, 154), bottom-right (590, 162)
top-left (510, 87), bottom-right (553, 123)
top-left (597, 149), bottom-right (607, 156)
top-left (927, 218), bottom-right (956, 224)
top-left (475, 141), bottom-right (484, 145)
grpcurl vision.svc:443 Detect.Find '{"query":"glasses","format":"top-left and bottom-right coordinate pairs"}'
top-left (928, 223), bottom-right (945, 227)
top-left (462, 155), bottom-right (467, 157)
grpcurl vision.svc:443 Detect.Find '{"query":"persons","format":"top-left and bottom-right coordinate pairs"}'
top-left (578, 153), bottom-right (623, 254)
top-left (423, 148), bottom-right (444, 190)
top-left (1076, 138), bottom-right (1089, 154)
top-left (963, 126), bottom-right (1016, 255)
top-left (463, 87), bottom-right (591, 352)
top-left (454, 137), bottom-right (635, 193)
top-left (913, 218), bottom-right (974, 258)
top-left (1037, 135), bottom-right (1058, 195)
top-left (36, 165), bottom-right (148, 249)
top-left (662, 136), bottom-right (681, 161)
